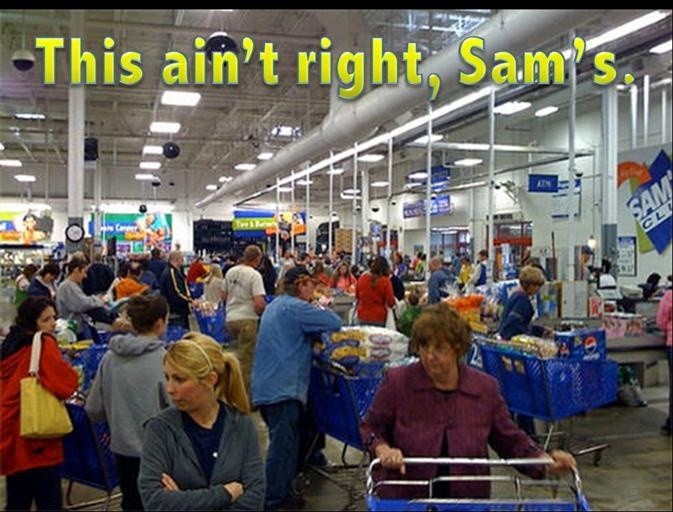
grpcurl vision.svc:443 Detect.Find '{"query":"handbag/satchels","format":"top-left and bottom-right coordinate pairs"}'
top-left (19, 375), bottom-right (74, 440)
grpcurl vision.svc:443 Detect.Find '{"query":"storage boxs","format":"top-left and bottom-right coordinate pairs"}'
top-left (553, 324), bottom-right (607, 365)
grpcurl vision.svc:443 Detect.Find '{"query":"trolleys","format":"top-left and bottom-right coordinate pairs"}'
top-left (474, 329), bottom-right (619, 467)
top-left (291, 352), bottom-right (399, 512)
top-left (81, 281), bottom-right (280, 345)
top-left (54, 348), bottom-right (133, 510)
top-left (358, 455), bottom-right (590, 512)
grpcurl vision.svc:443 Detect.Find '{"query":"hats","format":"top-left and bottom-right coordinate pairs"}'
top-left (286, 267), bottom-right (321, 284)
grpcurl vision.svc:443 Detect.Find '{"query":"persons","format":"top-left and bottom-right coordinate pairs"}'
top-left (85, 294), bottom-right (184, 512)
top-left (360, 303), bottom-right (577, 507)
top-left (0, 297), bottom-right (68, 510)
top-left (499, 265), bottom-right (552, 337)
top-left (137, 329), bottom-right (270, 510)
top-left (251, 262), bottom-right (342, 504)
top-left (0, 230), bottom-right (671, 437)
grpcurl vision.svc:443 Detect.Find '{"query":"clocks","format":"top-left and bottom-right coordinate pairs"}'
top-left (65, 223), bottom-right (86, 244)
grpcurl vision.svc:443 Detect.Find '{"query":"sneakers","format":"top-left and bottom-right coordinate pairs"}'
top-left (266, 495), bottom-right (304, 511)
top-left (324, 458), bottom-right (340, 472)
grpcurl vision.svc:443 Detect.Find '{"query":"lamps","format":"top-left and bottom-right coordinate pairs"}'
top-left (588, 144), bottom-right (597, 252)
